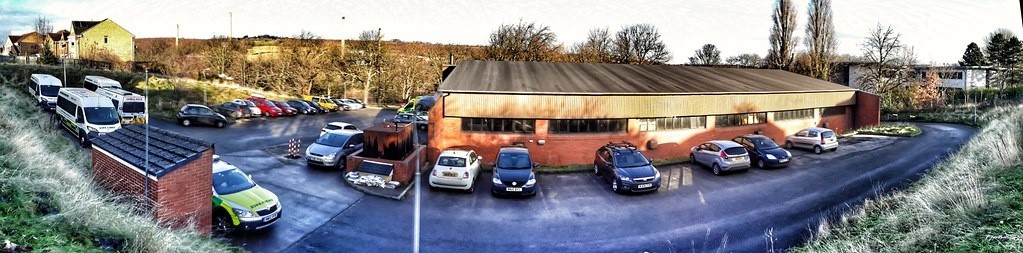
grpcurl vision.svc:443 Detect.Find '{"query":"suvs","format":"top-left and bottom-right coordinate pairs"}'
top-left (594, 141), bottom-right (661, 195)
top-left (488, 145), bottom-right (539, 198)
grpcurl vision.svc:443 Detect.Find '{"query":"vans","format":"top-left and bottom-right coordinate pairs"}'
top-left (303, 96), bottom-right (338, 111)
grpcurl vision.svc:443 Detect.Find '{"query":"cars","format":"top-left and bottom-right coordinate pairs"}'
top-left (320, 121), bottom-right (362, 138)
top-left (786, 127), bottom-right (838, 155)
top-left (331, 98), bottom-right (366, 111)
top-left (234, 99), bottom-right (261, 117)
top-left (690, 139), bottom-right (750, 177)
top-left (395, 114), bottom-right (428, 130)
top-left (247, 96), bottom-right (282, 117)
top-left (286, 99), bottom-right (316, 114)
top-left (210, 101), bottom-right (252, 119)
top-left (430, 148), bottom-right (482, 194)
top-left (303, 100), bottom-right (329, 114)
top-left (270, 100), bottom-right (298, 117)
top-left (175, 103), bottom-right (227, 129)
top-left (731, 134), bottom-right (792, 170)
top-left (304, 128), bottom-right (365, 170)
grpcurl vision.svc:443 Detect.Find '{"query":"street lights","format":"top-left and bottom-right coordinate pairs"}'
top-left (61, 48), bottom-right (66, 87)
top-left (145, 67), bottom-right (153, 215)
top-left (204, 69), bottom-right (210, 106)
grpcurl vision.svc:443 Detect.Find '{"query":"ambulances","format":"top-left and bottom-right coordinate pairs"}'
top-left (94, 87), bottom-right (146, 127)
top-left (55, 87), bottom-right (122, 149)
top-left (29, 73), bottom-right (63, 110)
top-left (83, 75), bottom-right (122, 92)
top-left (211, 153), bottom-right (283, 234)
top-left (395, 94), bottom-right (434, 117)
